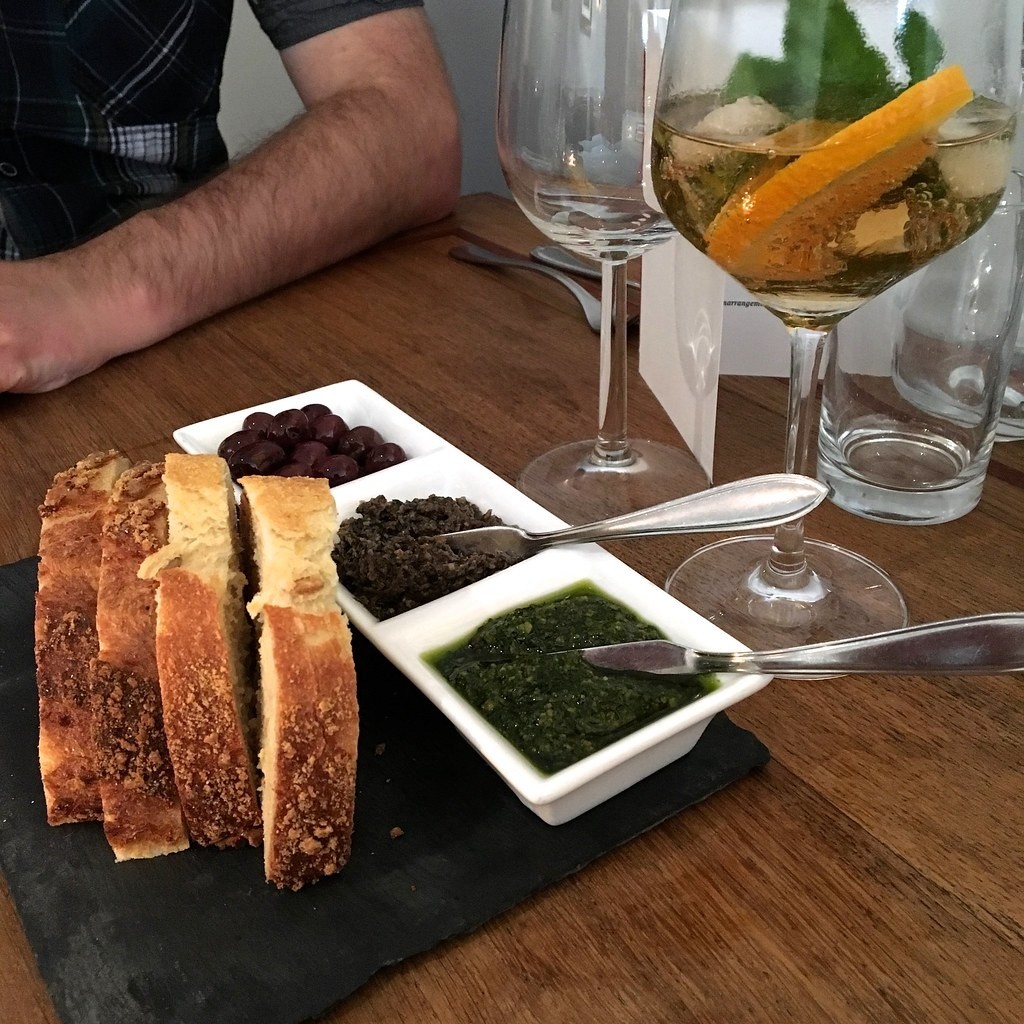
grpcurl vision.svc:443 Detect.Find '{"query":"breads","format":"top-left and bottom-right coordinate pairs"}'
top-left (29, 448), bottom-right (360, 894)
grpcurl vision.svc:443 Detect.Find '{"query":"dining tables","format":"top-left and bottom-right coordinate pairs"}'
top-left (0, 190), bottom-right (1024, 1024)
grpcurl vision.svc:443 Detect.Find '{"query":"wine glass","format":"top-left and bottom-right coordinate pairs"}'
top-left (495, 0), bottom-right (715, 543)
top-left (648, 0), bottom-right (1024, 679)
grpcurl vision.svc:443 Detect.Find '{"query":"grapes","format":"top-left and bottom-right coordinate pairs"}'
top-left (216, 404), bottom-right (405, 489)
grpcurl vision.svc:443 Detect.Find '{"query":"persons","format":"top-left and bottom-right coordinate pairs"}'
top-left (1, 0), bottom-right (465, 392)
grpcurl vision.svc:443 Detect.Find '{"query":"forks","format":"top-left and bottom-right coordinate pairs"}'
top-left (544, 612), bottom-right (1024, 679)
top-left (431, 474), bottom-right (828, 554)
top-left (449, 245), bottom-right (641, 334)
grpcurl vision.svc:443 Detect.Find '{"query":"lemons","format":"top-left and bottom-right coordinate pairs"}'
top-left (704, 68), bottom-right (974, 287)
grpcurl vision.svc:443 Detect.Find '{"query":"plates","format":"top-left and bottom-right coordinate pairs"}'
top-left (0, 554), bottom-right (772, 1024)
top-left (172, 379), bottom-right (775, 825)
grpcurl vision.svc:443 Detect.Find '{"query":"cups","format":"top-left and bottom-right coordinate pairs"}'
top-left (814, 169), bottom-right (1024, 525)
top-left (891, 202), bottom-right (1024, 443)
